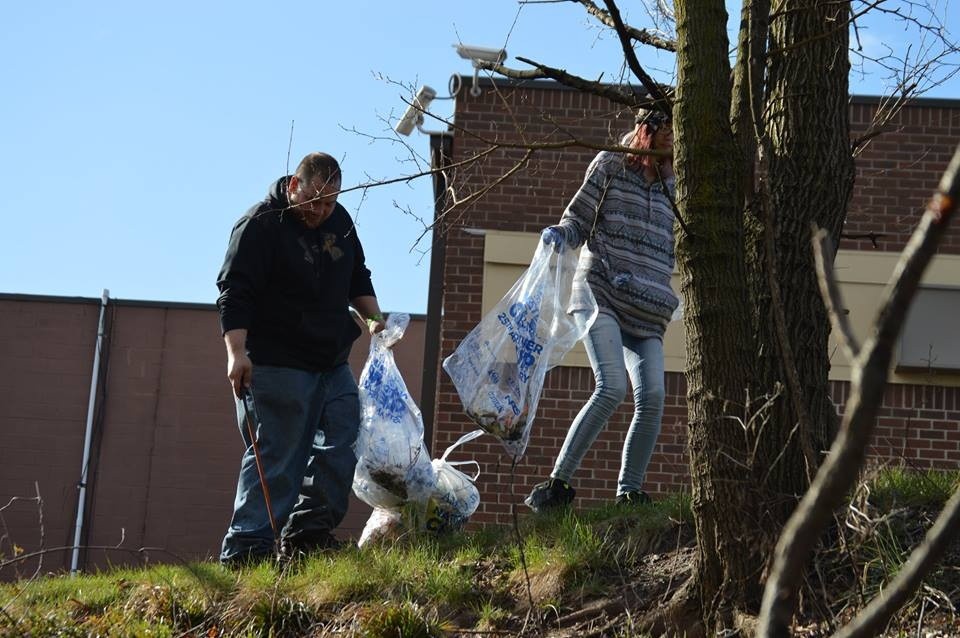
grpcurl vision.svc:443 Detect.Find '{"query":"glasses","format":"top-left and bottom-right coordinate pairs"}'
top-left (649, 122), bottom-right (673, 136)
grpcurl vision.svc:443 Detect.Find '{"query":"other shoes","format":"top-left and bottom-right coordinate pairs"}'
top-left (528, 477), bottom-right (575, 513)
top-left (616, 491), bottom-right (656, 508)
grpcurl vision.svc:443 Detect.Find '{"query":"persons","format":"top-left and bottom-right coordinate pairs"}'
top-left (524, 85), bottom-right (679, 514)
top-left (216, 151), bottom-right (385, 578)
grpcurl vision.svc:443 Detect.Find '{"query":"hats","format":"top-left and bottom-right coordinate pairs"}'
top-left (634, 86), bottom-right (679, 123)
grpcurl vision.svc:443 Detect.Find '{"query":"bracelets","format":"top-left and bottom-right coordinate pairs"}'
top-left (367, 312), bottom-right (383, 329)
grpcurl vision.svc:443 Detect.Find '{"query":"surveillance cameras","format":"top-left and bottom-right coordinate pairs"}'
top-left (456, 43), bottom-right (507, 63)
top-left (395, 86), bottom-right (434, 136)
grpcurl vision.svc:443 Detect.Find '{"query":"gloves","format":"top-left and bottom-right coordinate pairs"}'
top-left (543, 227), bottom-right (564, 256)
